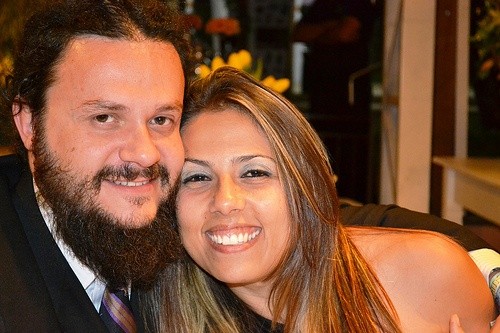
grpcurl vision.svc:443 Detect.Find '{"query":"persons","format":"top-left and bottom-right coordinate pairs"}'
top-left (176, 66), bottom-right (495, 333)
top-left (178, 0), bottom-right (500, 179)
top-left (0, 0), bottom-right (500, 333)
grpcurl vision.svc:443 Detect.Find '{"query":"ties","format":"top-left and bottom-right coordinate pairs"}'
top-left (100, 280), bottom-right (136, 333)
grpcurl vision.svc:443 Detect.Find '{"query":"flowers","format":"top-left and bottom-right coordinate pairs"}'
top-left (182, 14), bottom-right (291, 95)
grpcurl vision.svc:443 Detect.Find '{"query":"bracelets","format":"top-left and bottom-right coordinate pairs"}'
top-left (486, 270), bottom-right (500, 310)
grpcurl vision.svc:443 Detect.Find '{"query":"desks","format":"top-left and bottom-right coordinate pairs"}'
top-left (432, 156), bottom-right (500, 226)
